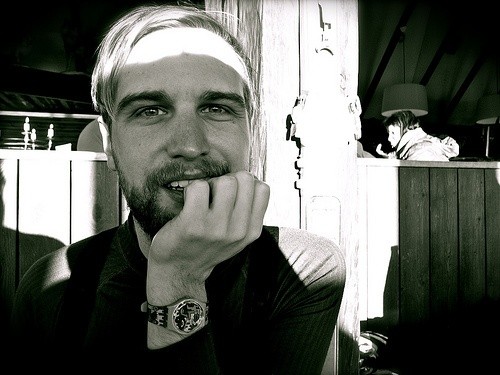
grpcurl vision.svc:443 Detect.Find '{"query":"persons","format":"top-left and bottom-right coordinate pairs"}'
top-left (384, 111), bottom-right (461, 162)
top-left (6, 5), bottom-right (348, 375)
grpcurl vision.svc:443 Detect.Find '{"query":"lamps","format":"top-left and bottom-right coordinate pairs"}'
top-left (378, 24), bottom-right (430, 118)
top-left (477, 63), bottom-right (500, 126)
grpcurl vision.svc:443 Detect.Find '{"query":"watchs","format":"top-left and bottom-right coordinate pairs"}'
top-left (139, 297), bottom-right (208, 335)
